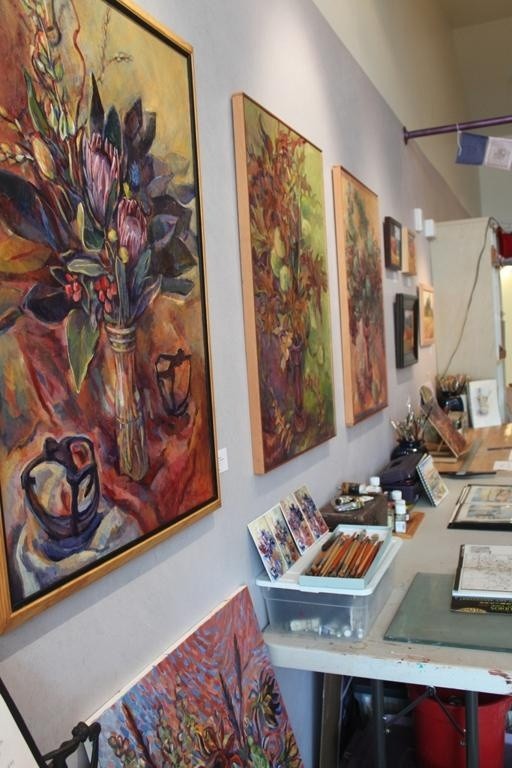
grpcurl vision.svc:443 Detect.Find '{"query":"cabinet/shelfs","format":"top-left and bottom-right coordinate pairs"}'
top-left (434, 215), bottom-right (506, 424)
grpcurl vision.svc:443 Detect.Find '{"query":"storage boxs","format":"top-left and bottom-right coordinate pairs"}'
top-left (254, 536), bottom-right (404, 641)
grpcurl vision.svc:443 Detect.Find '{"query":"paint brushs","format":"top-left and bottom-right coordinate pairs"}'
top-left (390, 397), bottom-right (433, 443)
top-left (438, 375), bottom-right (470, 394)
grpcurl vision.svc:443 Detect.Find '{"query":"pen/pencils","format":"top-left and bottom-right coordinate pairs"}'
top-left (305, 529), bottom-right (383, 578)
top-left (489, 447), bottom-right (510, 450)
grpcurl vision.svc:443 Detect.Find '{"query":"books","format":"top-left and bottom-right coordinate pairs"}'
top-left (414, 452), bottom-right (450, 508)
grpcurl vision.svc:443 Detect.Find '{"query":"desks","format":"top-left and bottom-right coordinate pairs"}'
top-left (261, 424), bottom-right (510, 768)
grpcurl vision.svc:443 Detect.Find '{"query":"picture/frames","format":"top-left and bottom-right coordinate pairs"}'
top-left (330, 162), bottom-right (388, 428)
top-left (0, 0), bottom-right (223, 635)
top-left (229, 92), bottom-right (339, 476)
top-left (384, 214), bottom-right (433, 368)
